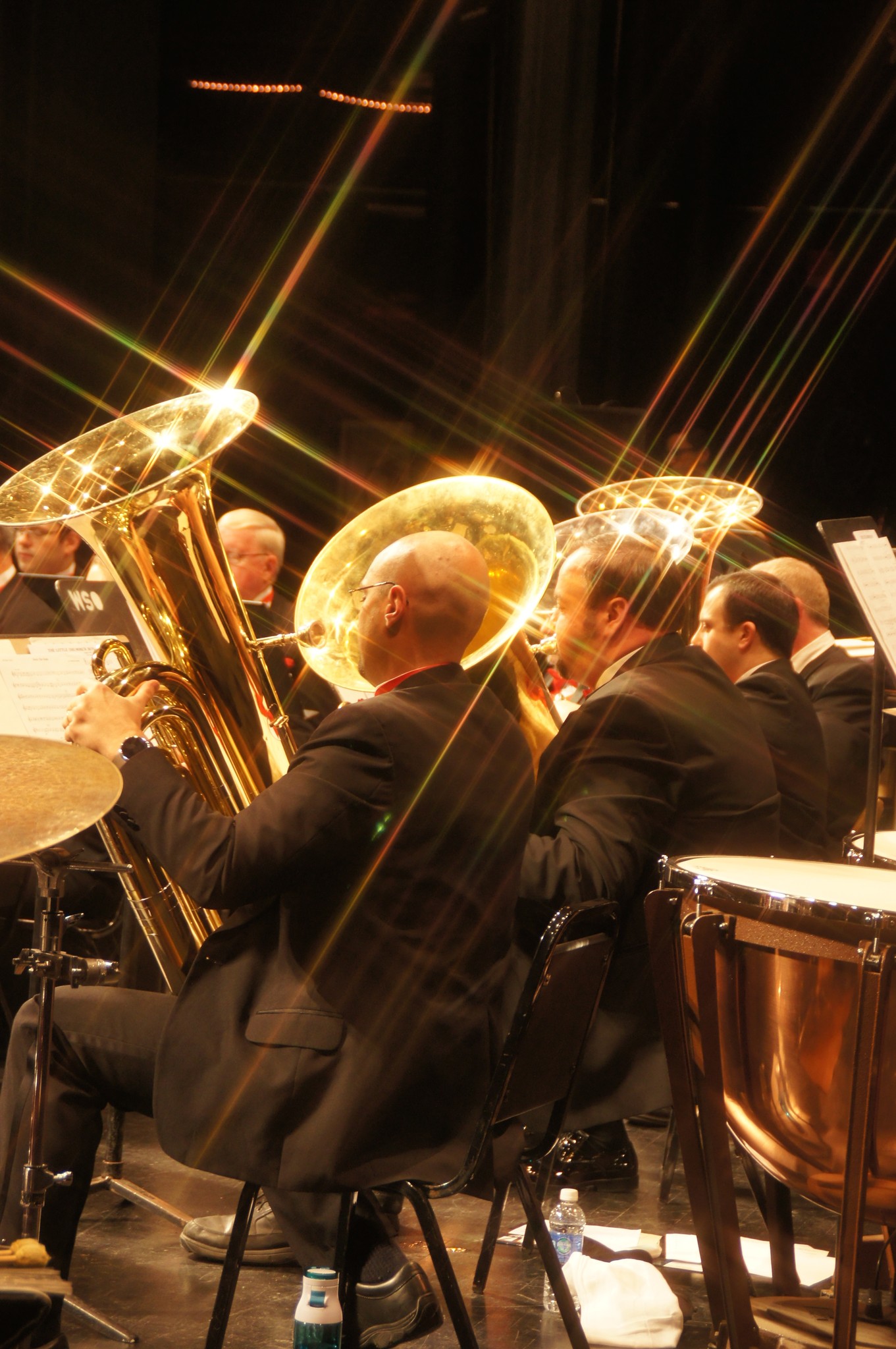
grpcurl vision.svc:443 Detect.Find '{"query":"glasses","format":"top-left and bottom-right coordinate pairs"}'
top-left (348, 581), bottom-right (409, 612)
top-left (16, 526), bottom-right (62, 537)
top-left (226, 552), bottom-right (269, 566)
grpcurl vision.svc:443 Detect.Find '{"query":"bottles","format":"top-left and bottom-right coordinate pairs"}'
top-left (293, 1268), bottom-right (343, 1349)
top-left (543, 1188), bottom-right (586, 1313)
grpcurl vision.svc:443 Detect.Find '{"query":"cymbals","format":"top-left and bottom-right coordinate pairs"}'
top-left (1, 732), bottom-right (124, 858)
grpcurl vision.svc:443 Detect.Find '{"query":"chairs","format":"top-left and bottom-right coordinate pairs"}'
top-left (207, 900), bottom-right (617, 1349)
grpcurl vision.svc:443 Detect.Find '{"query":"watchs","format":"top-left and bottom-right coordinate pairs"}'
top-left (114, 736), bottom-right (154, 766)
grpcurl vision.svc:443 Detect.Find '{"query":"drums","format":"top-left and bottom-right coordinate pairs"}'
top-left (665, 857), bottom-right (894, 1225)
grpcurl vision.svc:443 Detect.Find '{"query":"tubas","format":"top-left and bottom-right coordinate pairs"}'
top-left (292, 472), bottom-right (567, 779)
top-left (0, 387), bottom-right (303, 1001)
top-left (556, 507), bottom-right (696, 667)
top-left (576, 477), bottom-right (765, 626)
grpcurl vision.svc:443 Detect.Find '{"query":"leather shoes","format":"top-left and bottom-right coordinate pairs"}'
top-left (178, 1193), bottom-right (297, 1266)
top-left (356, 1260), bottom-right (444, 1349)
top-left (527, 1128), bottom-right (639, 1195)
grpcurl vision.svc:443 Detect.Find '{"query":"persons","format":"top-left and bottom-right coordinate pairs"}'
top-left (0, 496), bottom-right (896, 1261)
top-left (0, 530), bottom-right (544, 1348)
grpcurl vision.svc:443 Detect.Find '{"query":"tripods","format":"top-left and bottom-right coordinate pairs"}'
top-left (0, 628), bottom-right (195, 1228)
